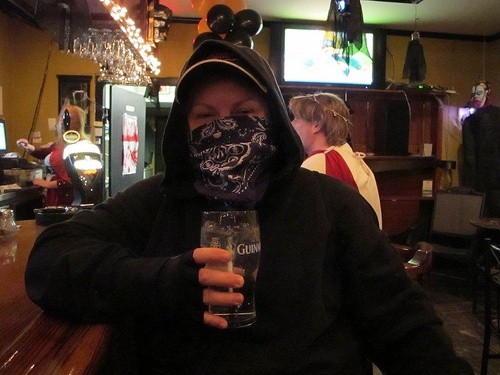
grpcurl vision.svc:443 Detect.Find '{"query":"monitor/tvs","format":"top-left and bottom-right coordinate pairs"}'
top-left (0, 120), bottom-right (7, 151)
top-left (270, 21), bottom-right (386, 90)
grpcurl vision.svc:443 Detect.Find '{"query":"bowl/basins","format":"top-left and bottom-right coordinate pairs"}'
top-left (35, 207), bottom-right (73, 226)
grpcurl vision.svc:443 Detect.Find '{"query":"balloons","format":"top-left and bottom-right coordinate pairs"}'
top-left (192, 0), bottom-right (263, 53)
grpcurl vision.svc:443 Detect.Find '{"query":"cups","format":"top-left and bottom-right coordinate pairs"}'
top-left (200, 210), bottom-right (261, 328)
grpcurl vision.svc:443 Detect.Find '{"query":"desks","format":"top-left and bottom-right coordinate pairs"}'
top-left (0, 220), bottom-right (116, 375)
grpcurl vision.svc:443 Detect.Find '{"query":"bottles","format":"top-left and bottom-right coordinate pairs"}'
top-left (0, 209), bottom-right (17, 242)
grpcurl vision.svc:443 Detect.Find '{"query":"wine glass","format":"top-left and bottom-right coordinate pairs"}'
top-left (72, 28), bottom-right (153, 85)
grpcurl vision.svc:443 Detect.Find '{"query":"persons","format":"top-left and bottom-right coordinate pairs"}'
top-left (289, 93), bottom-right (382, 233)
top-left (25, 41), bottom-right (477, 375)
top-left (15, 105), bottom-right (88, 211)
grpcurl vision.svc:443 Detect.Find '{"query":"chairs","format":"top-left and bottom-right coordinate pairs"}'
top-left (471, 230), bottom-right (500, 353)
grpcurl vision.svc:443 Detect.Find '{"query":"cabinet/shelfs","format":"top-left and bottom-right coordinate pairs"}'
top-left (281, 86), bottom-right (444, 239)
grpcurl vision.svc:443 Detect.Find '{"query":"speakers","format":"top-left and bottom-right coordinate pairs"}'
top-left (430, 187), bottom-right (487, 239)
